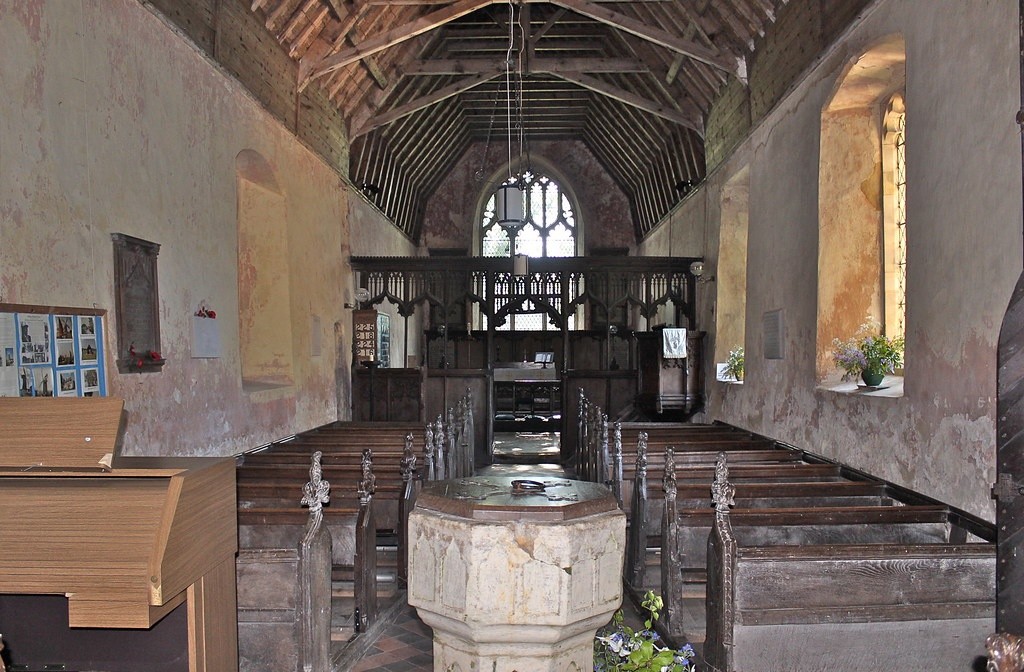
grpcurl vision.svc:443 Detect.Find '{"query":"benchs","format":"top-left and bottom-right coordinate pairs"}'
top-left (236, 387), bottom-right (474, 670)
top-left (579, 387), bottom-right (997, 671)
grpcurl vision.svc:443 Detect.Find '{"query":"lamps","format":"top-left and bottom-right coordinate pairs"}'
top-left (496, 2), bottom-right (524, 229)
top-left (690, 261), bottom-right (715, 284)
top-left (343, 288), bottom-right (370, 308)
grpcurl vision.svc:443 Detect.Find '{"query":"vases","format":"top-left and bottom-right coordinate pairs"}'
top-left (862, 369), bottom-right (884, 386)
top-left (735, 370), bottom-right (743, 381)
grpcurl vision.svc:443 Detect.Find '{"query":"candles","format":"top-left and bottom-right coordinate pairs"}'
top-left (468, 322), bottom-right (471, 335)
top-left (369, 353), bottom-right (373, 361)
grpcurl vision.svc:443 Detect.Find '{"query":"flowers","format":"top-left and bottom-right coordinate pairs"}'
top-left (194, 308), bottom-right (218, 319)
top-left (720, 343), bottom-right (744, 380)
top-left (127, 343), bottom-right (143, 368)
top-left (830, 315), bottom-right (905, 385)
top-left (145, 349), bottom-right (160, 360)
